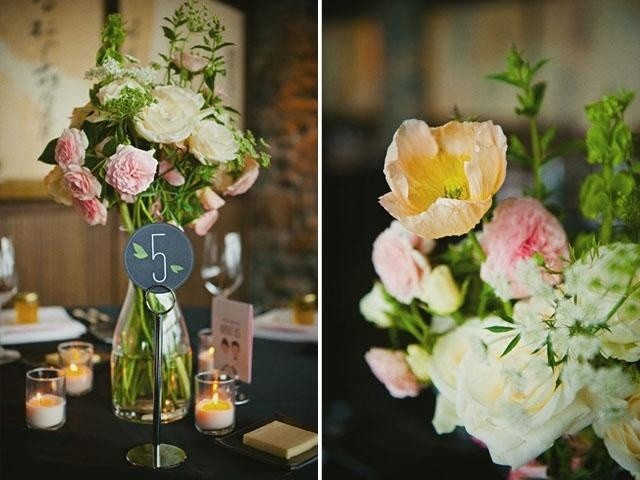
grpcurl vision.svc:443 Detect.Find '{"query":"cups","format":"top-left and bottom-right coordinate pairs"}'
top-left (22, 341), bottom-right (93, 433)
top-left (193, 368), bottom-right (235, 439)
top-left (196, 327), bottom-right (218, 373)
top-left (14, 292), bottom-right (39, 326)
top-left (293, 290), bottom-right (319, 326)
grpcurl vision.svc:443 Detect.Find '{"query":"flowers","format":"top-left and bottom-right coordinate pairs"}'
top-left (34, 0), bottom-right (274, 412)
top-left (360, 35), bottom-right (640, 480)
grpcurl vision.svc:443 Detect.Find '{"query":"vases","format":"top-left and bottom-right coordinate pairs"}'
top-left (108, 281), bottom-right (194, 424)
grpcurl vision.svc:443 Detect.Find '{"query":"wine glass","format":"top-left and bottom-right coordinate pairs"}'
top-left (196, 228), bottom-right (245, 341)
top-left (0, 235), bottom-right (21, 364)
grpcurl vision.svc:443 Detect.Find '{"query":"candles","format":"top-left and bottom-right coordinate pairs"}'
top-left (27, 366), bottom-right (92, 426)
top-left (195, 399), bottom-right (235, 431)
